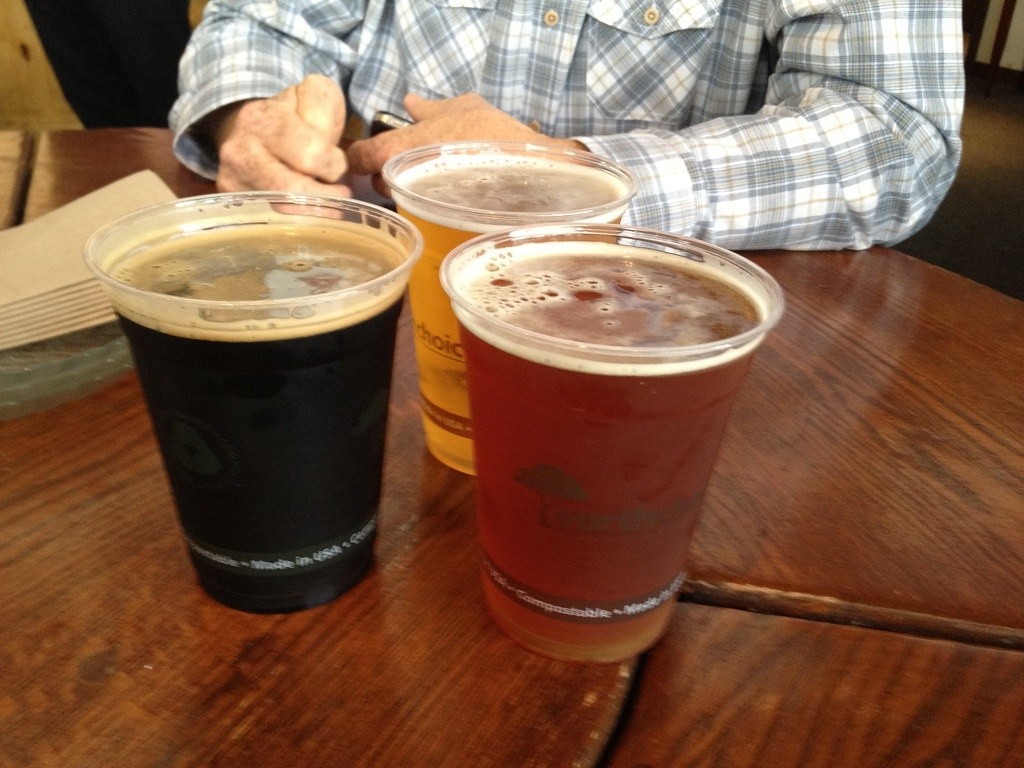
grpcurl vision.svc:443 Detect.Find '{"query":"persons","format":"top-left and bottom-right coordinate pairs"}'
top-left (166, 0), bottom-right (969, 257)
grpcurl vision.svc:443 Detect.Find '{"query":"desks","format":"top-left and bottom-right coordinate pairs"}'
top-left (0, 126), bottom-right (1023, 766)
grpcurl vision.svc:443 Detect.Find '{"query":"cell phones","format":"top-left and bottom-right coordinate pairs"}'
top-left (370, 110), bottom-right (416, 137)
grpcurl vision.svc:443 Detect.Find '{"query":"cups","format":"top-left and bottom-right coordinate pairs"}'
top-left (434, 223), bottom-right (784, 664)
top-left (82, 188), bottom-right (425, 613)
top-left (381, 144), bottom-right (640, 475)
